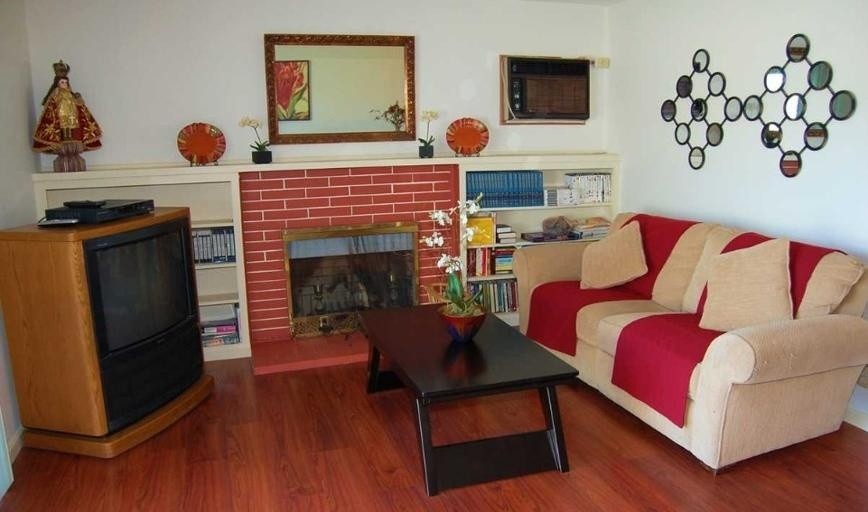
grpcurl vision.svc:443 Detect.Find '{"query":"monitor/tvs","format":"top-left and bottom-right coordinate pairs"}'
top-left (82, 217), bottom-right (199, 363)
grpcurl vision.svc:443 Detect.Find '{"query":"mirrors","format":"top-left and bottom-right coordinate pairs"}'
top-left (264, 33), bottom-right (415, 146)
top-left (660, 30), bottom-right (856, 178)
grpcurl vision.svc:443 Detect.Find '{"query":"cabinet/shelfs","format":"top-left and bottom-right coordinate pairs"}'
top-left (458, 155), bottom-right (620, 327)
top-left (31, 166), bottom-right (253, 365)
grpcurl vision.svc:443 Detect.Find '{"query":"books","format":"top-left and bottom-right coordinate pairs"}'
top-left (193, 228), bottom-right (237, 264)
top-left (466, 172), bottom-right (612, 312)
top-left (199, 304), bottom-right (243, 346)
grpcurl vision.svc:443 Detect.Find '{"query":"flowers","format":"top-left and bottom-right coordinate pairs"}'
top-left (369, 99), bottom-right (406, 133)
top-left (419, 193), bottom-right (487, 312)
top-left (418, 108), bottom-right (439, 144)
top-left (239, 114), bottom-right (270, 150)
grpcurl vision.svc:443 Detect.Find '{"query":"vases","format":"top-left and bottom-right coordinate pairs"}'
top-left (419, 146), bottom-right (434, 158)
top-left (251, 151), bottom-right (272, 163)
top-left (438, 301), bottom-right (486, 341)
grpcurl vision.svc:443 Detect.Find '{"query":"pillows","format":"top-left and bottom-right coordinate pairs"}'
top-left (579, 220), bottom-right (650, 291)
top-left (698, 236), bottom-right (794, 333)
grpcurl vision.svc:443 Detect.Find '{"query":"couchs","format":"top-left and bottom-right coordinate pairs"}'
top-left (504, 213), bottom-right (868, 481)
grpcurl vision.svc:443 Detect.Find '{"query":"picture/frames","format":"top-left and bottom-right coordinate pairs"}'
top-left (272, 60), bottom-right (309, 119)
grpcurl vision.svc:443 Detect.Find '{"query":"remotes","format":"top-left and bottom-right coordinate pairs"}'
top-left (35, 219), bottom-right (80, 228)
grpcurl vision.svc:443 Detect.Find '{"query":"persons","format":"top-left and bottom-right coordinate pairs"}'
top-left (32, 58), bottom-right (105, 171)
